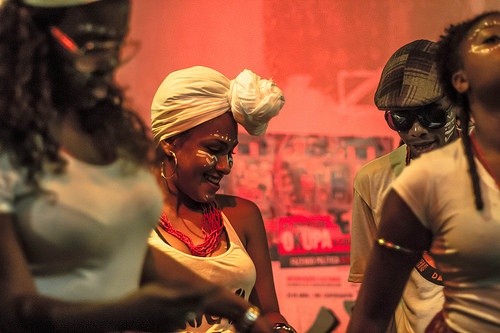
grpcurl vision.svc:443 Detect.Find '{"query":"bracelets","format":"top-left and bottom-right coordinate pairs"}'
top-left (235, 303), bottom-right (260, 333)
top-left (272, 321), bottom-right (301, 333)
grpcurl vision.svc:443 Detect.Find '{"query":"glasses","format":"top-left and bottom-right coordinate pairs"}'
top-left (47, 24), bottom-right (142, 75)
top-left (385, 100), bottom-right (456, 133)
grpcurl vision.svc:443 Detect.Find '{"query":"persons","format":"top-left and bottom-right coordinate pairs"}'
top-left (1, 1), bottom-right (272, 333)
top-left (346, 9), bottom-right (500, 333)
top-left (135, 65), bottom-right (301, 333)
top-left (347, 36), bottom-right (468, 333)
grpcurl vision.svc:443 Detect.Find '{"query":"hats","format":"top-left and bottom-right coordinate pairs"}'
top-left (374, 39), bottom-right (445, 111)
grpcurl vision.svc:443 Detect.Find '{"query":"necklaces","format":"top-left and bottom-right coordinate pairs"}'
top-left (156, 197), bottom-right (225, 258)
